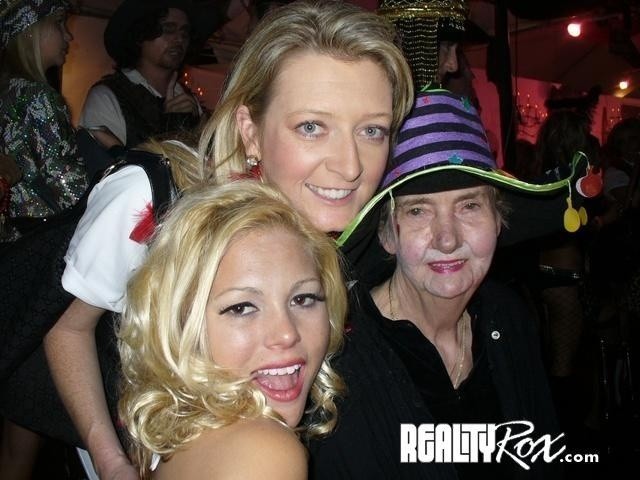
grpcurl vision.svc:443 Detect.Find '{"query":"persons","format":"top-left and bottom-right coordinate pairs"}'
top-left (1, 1), bottom-right (94, 243)
top-left (301, 89), bottom-right (589, 480)
top-left (44, 1), bottom-right (419, 480)
top-left (77, 0), bottom-right (205, 158)
top-left (377, 2), bottom-right (640, 416)
top-left (113, 178), bottom-right (347, 480)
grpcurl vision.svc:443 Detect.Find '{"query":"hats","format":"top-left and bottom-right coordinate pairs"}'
top-left (332, 90), bottom-right (571, 248)
top-left (0, 0), bottom-right (73, 51)
top-left (104, 1), bottom-right (222, 62)
